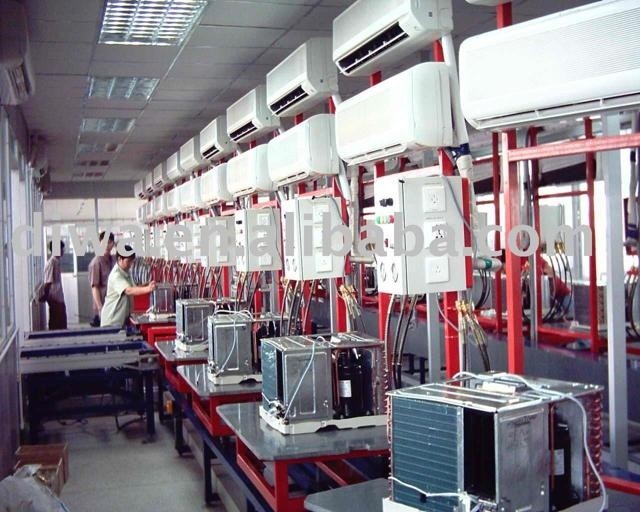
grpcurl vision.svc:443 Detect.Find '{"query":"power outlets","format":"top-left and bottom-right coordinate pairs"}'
top-left (258, 214), bottom-right (273, 266)
top-left (422, 185), bottom-right (451, 284)
top-left (313, 205), bottom-right (333, 272)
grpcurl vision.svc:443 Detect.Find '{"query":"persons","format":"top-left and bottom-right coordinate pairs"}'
top-left (39, 239), bottom-right (67, 330)
top-left (89, 230), bottom-right (158, 326)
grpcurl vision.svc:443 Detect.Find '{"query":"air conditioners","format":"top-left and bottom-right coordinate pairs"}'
top-left (137, 4), bottom-right (640, 226)
